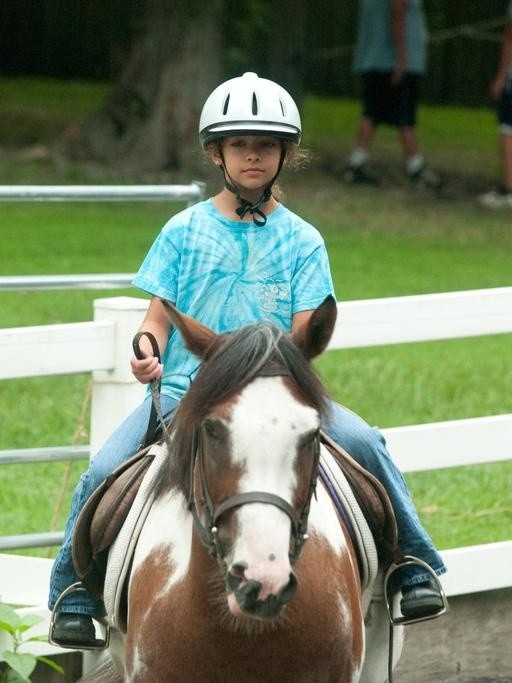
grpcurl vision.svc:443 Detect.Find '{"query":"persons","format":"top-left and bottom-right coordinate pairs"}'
top-left (337, 1), bottom-right (449, 192)
top-left (49, 70), bottom-right (447, 648)
top-left (474, 11), bottom-right (512, 210)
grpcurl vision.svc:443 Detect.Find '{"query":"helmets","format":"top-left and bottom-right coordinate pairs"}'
top-left (196, 71), bottom-right (305, 153)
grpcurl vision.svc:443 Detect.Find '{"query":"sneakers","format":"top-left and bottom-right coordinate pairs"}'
top-left (340, 163), bottom-right (512, 210)
top-left (400, 573), bottom-right (446, 617)
top-left (48, 610), bottom-right (111, 651)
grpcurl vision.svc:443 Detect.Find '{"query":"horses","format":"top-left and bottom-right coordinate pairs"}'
top-left (82, 295), bottom-right (409, 683)
